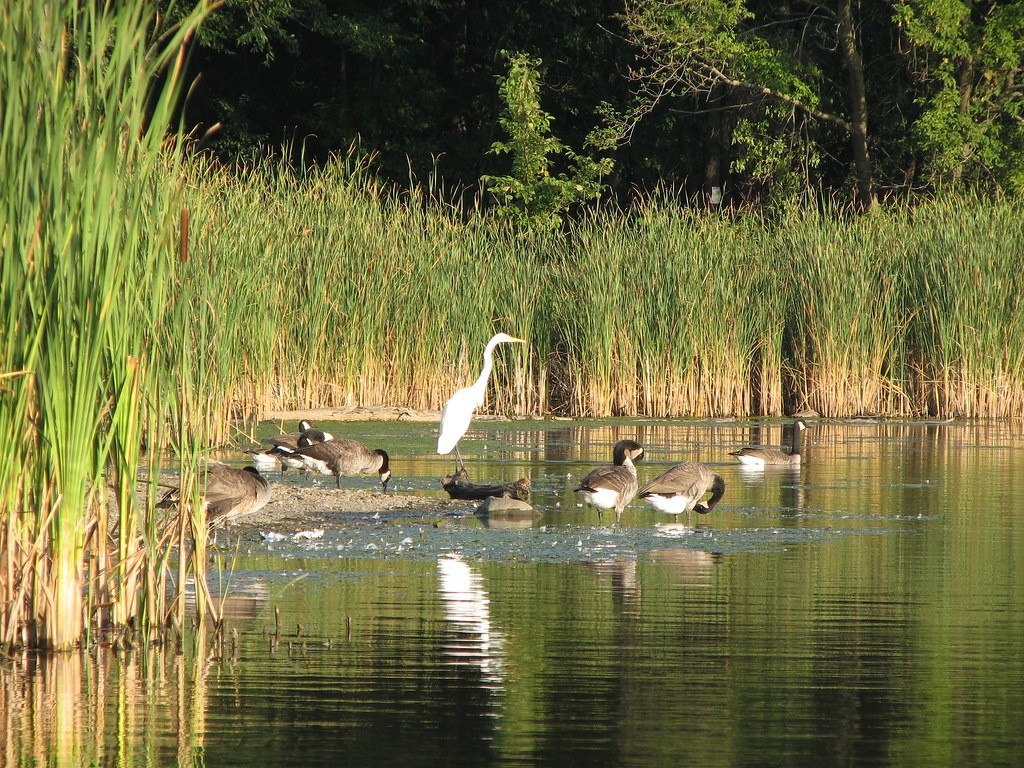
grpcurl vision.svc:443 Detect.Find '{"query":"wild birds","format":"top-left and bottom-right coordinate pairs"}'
top-left (155, 466), bottom-right (270, 528)
top-left (573, 440), bottom-right (644, 525)
top-left (437, 333), bottom-right (530, 480)
top-left (244, 417), bottom-right (391, 492)
top-left (726, 419), bottom-right (815, 465)
top-left (636, 461), bottom-right (726, 523)
